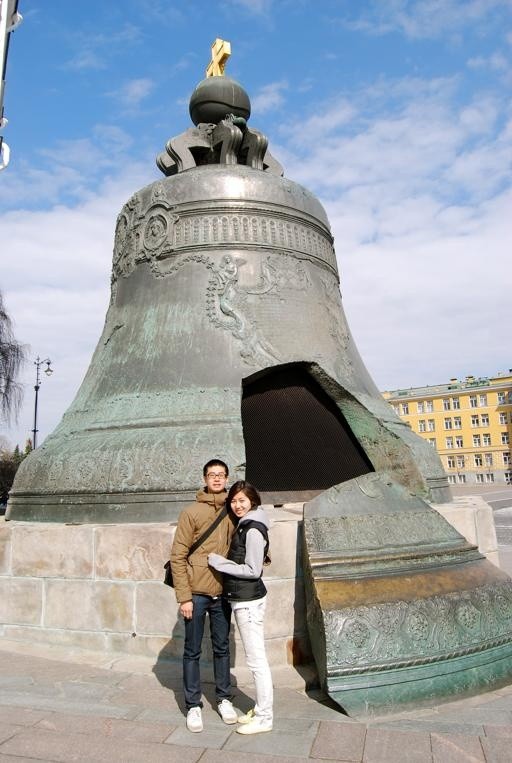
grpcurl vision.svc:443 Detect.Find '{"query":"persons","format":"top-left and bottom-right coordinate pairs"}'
top-left (170, 458), bottom-right (271, 734)
top-left (209, 482), bottom-right (274, 736)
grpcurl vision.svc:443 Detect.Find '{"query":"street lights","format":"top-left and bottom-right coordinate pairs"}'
top-left (32, 356), bottom-right (54, 450)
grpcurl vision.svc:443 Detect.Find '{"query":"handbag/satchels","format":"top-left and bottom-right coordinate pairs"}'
top-left (163, 560), bottom-right (175, 588)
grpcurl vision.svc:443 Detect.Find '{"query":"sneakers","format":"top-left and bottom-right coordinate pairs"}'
top-left (187, 706), bottom-right (203, 733)
top-left (217, 699), bottom-right (238, 724)
top-left (238, 708), bottom-right (257, 723)
top-left (236, 723), bottom-right (273, 735)
